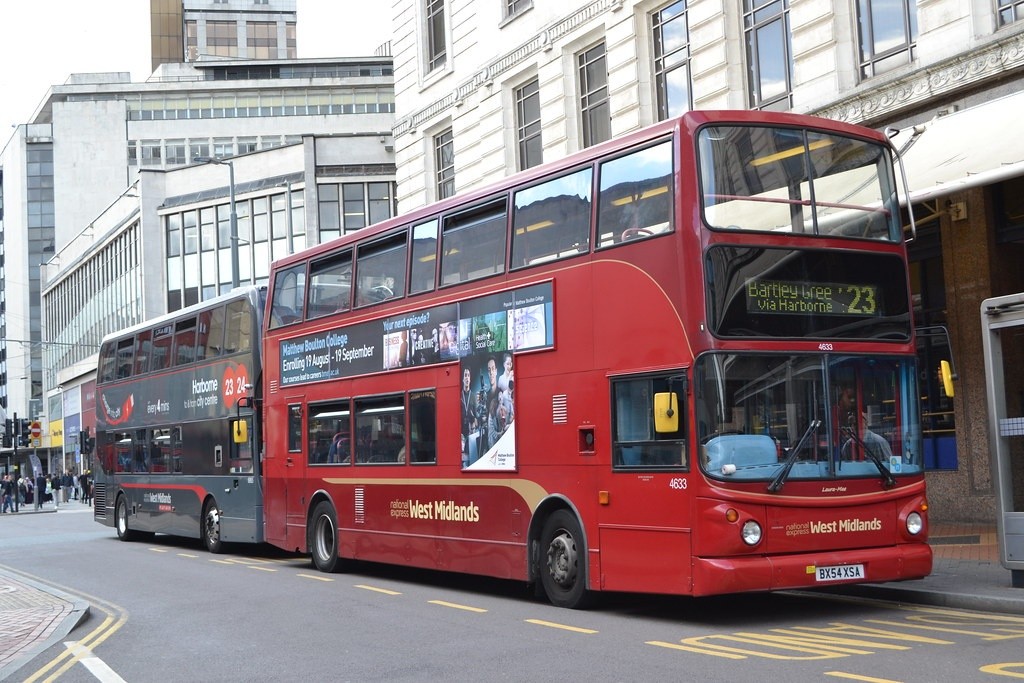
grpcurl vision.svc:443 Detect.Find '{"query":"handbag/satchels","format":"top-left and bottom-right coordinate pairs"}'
top-left (0, 489), bottom-right (6, 495)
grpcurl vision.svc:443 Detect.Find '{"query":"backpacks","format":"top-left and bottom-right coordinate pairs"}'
top-left (18, 480), bottom-right (26, 494)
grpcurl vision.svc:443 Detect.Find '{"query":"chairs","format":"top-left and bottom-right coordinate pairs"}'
top-left (295, 430), bottom-right (423, 462)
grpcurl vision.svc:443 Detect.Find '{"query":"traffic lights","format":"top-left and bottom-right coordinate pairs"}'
top-left (80, 431), bottom-right (89, 455)
top-left (1, 419), bottom-right (13, 446)
top-left (22, 419), bottom-right (32, 446)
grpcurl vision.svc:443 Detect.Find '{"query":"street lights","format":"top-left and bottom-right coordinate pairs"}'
top-left (195, 155), bottom-right (239, 291)
top-left (57, 386), bottom-right (65, 475)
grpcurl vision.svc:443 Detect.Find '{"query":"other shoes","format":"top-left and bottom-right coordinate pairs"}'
top-left (55, 506), bottom-right (58, 509)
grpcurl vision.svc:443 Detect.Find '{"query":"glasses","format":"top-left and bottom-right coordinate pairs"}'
top-left (848, 411), bottom-right (866, 421)
top-left (432, 333), bottom-right (436, 337)
top-left (413, 330), bottom-right (417, 334)
top-left (438, 325), bottom-right (456, 342)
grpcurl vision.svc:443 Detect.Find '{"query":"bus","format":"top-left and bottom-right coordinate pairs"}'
top-left (262, 108), bottom-right (962, 609)
top-left (89, 284), bottom-right (356, 554)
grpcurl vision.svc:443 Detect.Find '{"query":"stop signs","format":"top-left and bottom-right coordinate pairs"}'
top-left (31, 424), bottom-right (41, 438)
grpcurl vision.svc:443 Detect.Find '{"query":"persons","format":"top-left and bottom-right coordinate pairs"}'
top-left (459, 353), bottom-right (514, 468)
top-left (843, 410), bottom-right (893, 462)
top-left (398, 444), bottom-right (417, 462)
top-left (410, 322), bottom-right (456, 365)
top-left (0, 470), bottom-right (93, 513)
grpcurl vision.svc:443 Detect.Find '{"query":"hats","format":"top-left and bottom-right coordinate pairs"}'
top-left (26, 477), bottom-right (29, 480)
